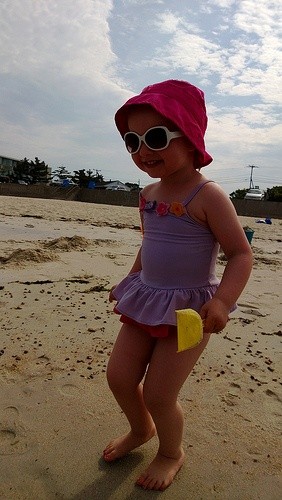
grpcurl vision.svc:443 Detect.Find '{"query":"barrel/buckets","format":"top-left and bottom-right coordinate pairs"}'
top-left (245, 231), bottom-right (254, 244)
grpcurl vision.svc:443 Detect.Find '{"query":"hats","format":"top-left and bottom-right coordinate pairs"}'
top-left (113, 80), bottom-right (214, 170)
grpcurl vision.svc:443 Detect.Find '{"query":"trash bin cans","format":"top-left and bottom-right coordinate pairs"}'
top-left (243, 229), bottom-right (253, 245)
top-left (63, 180), bottom-right (69, 187)
top-left (87, 181), bottom-right (95, 189)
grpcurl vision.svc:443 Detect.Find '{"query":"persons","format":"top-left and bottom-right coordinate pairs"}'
top-left (103, 78), bottom-right (254, 492)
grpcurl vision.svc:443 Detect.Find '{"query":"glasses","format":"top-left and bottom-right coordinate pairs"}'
top-left (124, 125), bottom-right (189, 154)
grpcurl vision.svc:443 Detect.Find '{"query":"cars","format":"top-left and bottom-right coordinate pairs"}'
top-left (0, 177), bottom-right (78, 187)
top-left (244, 188), bottom-right (265, 201)
top-left (106, 185), bottom-right (127, 191)
